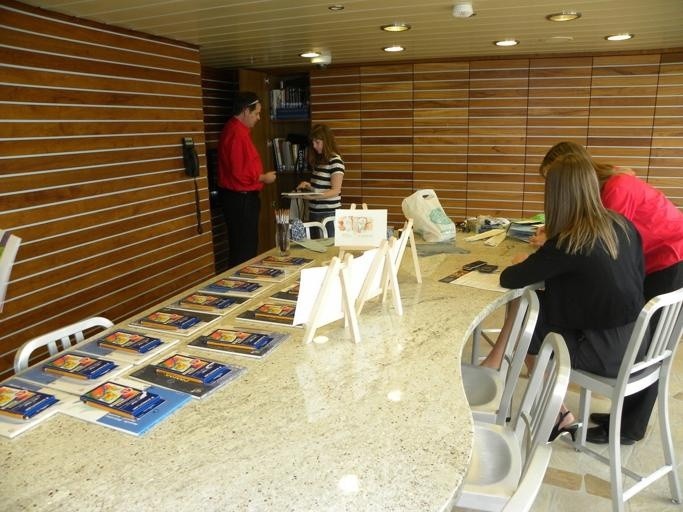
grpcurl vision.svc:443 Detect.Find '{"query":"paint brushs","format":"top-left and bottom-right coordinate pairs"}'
top-left (275, 209), bottom-right (289, 252)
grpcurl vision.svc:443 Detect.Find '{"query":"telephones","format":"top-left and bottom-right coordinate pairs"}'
top-left (183, 148), bottom-right (199, 177)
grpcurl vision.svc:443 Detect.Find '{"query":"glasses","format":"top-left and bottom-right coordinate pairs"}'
top-left (246, 96), bottom-right (262, 108)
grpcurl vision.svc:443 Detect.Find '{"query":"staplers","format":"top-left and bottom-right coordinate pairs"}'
top-left (479, 224), bottom-right (498, 233)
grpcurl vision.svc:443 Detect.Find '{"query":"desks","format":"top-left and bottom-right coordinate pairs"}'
top-left (0, 227), bottom-right (544, 512)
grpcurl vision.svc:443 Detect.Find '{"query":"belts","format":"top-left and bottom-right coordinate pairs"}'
top-left (238, 191), bottom-right (258, 195)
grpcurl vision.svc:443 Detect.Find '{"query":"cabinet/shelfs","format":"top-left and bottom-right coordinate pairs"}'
top-left (236, 66), bottom-right (311, 256)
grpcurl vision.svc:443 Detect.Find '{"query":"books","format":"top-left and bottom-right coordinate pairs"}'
top-left (281, 188), bottom-right (324, 200)
top-left (270, 78), bottom-right (309, 174)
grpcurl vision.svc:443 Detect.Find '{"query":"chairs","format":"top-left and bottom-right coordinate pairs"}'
top-left (454, 287), bottom-right (683, 512)
top-left (300, 220), bottom-right (328, 239)
top-left (11, 314), bottom-right (115, 374)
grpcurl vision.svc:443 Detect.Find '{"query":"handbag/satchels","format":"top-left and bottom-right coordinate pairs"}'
top-left (401, 189), bottom-right (457, 243)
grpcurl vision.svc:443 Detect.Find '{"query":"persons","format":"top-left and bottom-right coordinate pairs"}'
top-left (294, 121), bottom-right (347, 240)
top-left (475, 149), bottom-right (645, 449)
top-left (526, 142), bottom-right (681, 446)
top-left (215, 91), bottom-right (279, 274)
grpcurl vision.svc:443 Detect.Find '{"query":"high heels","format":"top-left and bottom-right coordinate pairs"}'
top-left (548, 408), bottom-right (578, 443)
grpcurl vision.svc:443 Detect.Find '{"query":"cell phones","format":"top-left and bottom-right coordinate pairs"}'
top-left (463, 261), bottom-right (487, 271)
top-left (479, 265), bottom-right (497, 273)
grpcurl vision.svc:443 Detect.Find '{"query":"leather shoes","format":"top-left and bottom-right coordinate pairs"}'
top-left (585, 413), bottom-right (635, 445)
